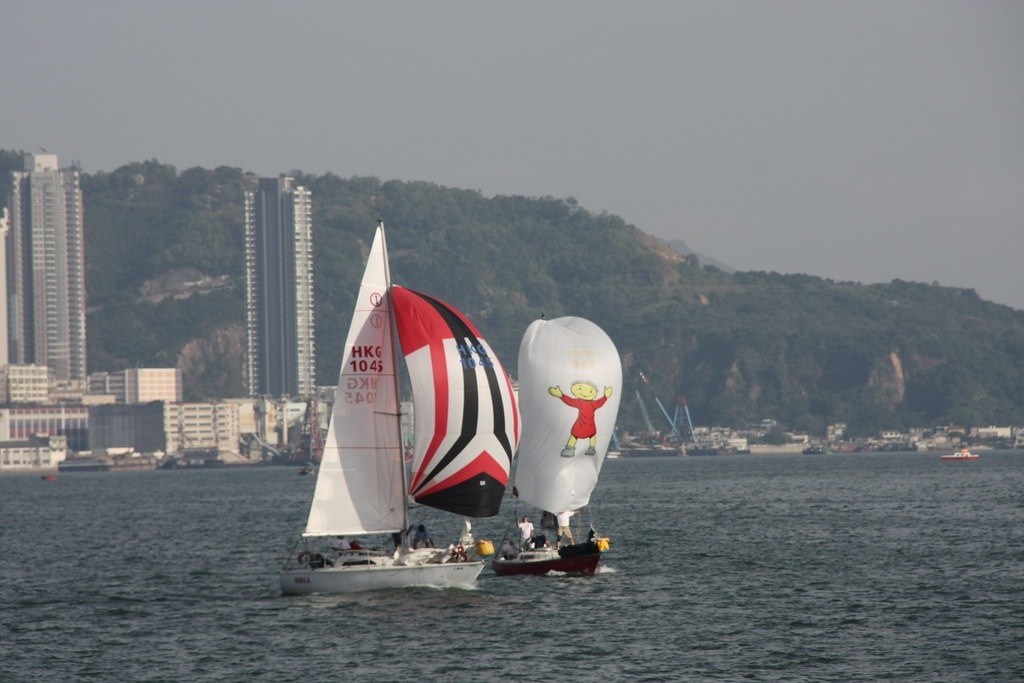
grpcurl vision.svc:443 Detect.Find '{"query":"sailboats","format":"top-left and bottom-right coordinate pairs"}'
top-left (488, 314), bottom-right (624, 575)
top-left (279, 217), bottom-right (526, 592)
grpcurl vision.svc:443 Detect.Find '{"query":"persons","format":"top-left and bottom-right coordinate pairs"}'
top-left (392, 533), bottom-right (402, 548)
top-left (405, 524), bottom-right (435, 550)
top-left (309, 535), bottom-right (362, 570)
top-left (516, 511), bottom-right (574, 551)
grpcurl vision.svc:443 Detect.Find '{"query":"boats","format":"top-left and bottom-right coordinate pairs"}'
top-left (619, 444), bottom-right (737, 457)
top-left (940, 451), bottom-right (980, 459)
